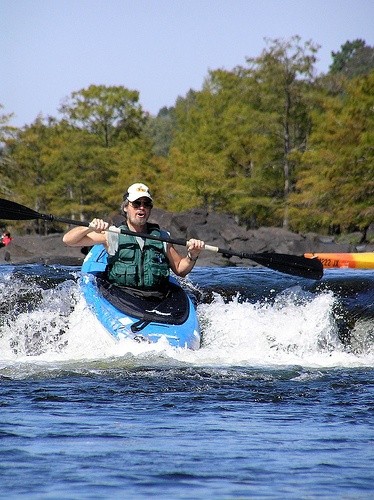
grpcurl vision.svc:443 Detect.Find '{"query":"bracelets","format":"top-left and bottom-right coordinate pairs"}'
top-left (187, 252), bottom-right (198, 261)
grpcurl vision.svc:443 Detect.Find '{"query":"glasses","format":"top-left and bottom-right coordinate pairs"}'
top-left (131, 200), bottom-right (154, 210)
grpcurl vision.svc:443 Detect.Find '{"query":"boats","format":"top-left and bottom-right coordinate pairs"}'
top-left (80, 243), bottom-right (202, 352)
top-left (302, 252), bottom-right (374, 268)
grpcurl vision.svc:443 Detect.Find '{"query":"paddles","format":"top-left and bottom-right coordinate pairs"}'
top-left (0, 196), bottom-right (323, 280)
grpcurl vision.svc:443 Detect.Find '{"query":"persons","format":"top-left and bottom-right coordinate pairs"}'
top-left (62, 180), bottom-right (205, 292)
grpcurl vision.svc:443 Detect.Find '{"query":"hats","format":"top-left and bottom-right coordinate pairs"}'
top-left (123, 182), bottom-right (153, 202)
